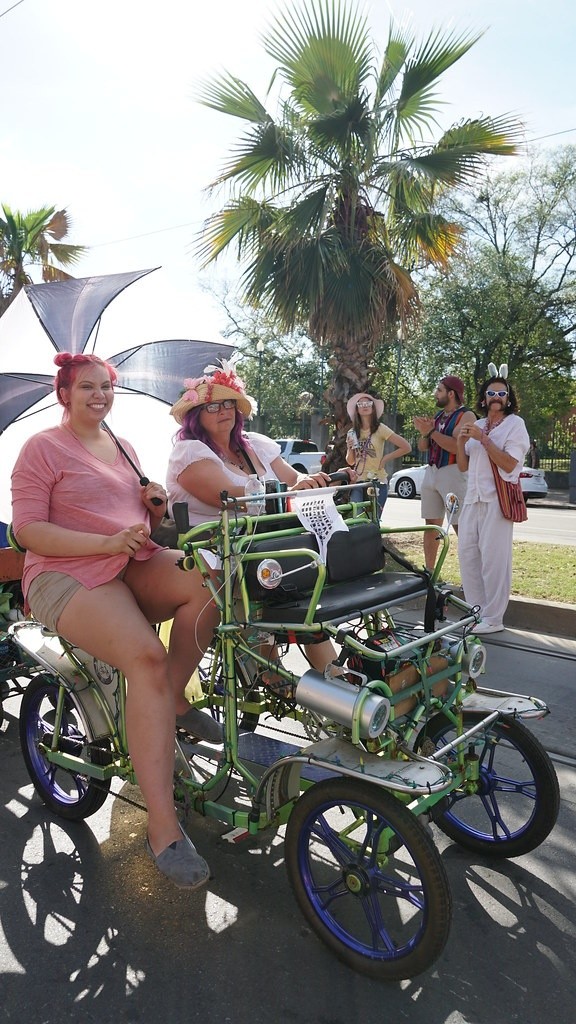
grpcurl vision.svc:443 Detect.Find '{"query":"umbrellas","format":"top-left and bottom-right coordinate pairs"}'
top-left (0, 265), bottom-right (239, 525)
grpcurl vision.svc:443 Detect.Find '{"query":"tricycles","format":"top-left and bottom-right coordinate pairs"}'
top-left (9, 479), bottom-right (561, 981)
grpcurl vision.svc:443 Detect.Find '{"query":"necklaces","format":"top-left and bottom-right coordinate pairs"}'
top-left (484, 416), bottom-right (504, 433)
top-left (355, 435), bottom-right (371, 475)
top-left (229, 456), bottom-right (244, 471)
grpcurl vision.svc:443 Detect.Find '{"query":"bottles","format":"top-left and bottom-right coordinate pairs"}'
top-left (244, 474), bottom-right (266, 516)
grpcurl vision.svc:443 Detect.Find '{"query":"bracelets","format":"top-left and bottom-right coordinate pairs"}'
top-left (427, 429), bottom-right (435, 438)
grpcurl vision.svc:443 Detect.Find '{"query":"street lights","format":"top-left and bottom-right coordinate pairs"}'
top-left (394, 327), bottom-right (406, 414)
top-left (320, 354), bottom-right (332, 406)
top-left (256, 338), bottom-right (264, 433)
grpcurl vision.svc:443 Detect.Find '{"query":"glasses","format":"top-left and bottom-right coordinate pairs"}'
top-left (201, 399), bottom-right (236, 414)
top-left (486, 390), bottom-right (508, 398)
top-left (356, 401), bottom-right (374, 408)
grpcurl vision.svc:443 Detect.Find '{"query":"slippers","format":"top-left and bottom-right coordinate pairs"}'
top-left (267, 677), bottom-right (297, 701)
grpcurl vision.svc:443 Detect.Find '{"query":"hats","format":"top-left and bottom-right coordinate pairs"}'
top-left (442, 376), bottom-right (465, 404)
top-left (346, 393), bottom-right (385, 422)
top-left (169, 374), bottom-right (252, 425)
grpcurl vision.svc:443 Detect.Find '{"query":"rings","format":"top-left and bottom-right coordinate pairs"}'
top-left (467, 429), bottom-right (469, 433)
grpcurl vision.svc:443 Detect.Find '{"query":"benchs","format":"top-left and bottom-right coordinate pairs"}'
top-left (235, 518), bottom-right (450, 631)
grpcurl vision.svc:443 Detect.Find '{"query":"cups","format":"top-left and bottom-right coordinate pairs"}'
top-left (278, 482), bottom-right (288, 513)
top-left (260, 479), bottom-right (281, 515)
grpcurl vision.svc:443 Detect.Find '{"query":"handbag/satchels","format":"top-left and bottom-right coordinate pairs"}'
top-left (494, 474), bottom-right (528, 524)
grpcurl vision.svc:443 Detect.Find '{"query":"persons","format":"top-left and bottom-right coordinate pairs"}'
top-left (413, 376), bottom-right (478, 575)
top-left (166, 368), bottom-right (358, 701)
top-left (456, 377), bottom-right (529, 634)
top-left (10, 353), bottom-right (226, 891)
top-left (345, 391), bottom-right (411, 519)
top-left (528, 441), bottom-right (541, 469)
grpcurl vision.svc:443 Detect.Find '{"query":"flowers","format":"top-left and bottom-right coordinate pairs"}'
top-left (202, 351), bottom-right (258, 422)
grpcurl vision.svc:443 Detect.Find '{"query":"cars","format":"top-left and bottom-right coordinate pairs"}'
top-left (390, 464), bottom-right (547, 505)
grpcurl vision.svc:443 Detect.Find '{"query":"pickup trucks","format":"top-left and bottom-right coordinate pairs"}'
top-left (272, 438), bottom-right (327, 475)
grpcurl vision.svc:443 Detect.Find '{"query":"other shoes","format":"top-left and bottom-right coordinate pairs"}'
top-left (469, 620), bottom-right (504, 634)
top-left (145, 820), bottom-right (209, 890)
top-left (173, 704), bottom-right (223, 745)
